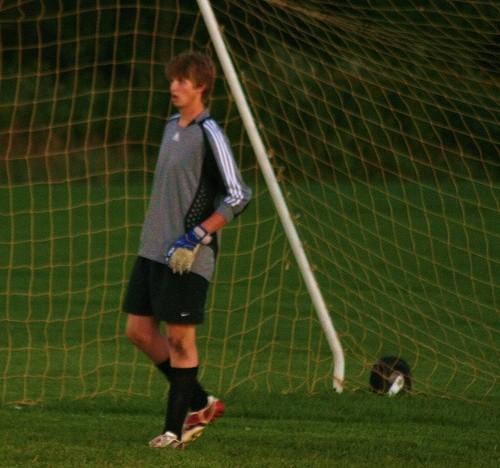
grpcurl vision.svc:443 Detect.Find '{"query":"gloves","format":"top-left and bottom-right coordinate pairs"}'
top-left (164, 223), bottom-right (212, 278)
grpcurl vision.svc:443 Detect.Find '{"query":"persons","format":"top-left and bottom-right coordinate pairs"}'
top-left (121, 51), bottom-right (252, 450)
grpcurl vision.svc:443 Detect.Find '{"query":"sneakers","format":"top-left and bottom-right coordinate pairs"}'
top-left (178, 394), bottom-right (224, 447)
top-left (142, 432), bottom-right (188, 450)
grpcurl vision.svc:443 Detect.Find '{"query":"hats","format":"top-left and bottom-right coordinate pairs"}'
top-left (367, 356), bottom-right (412, 397)
